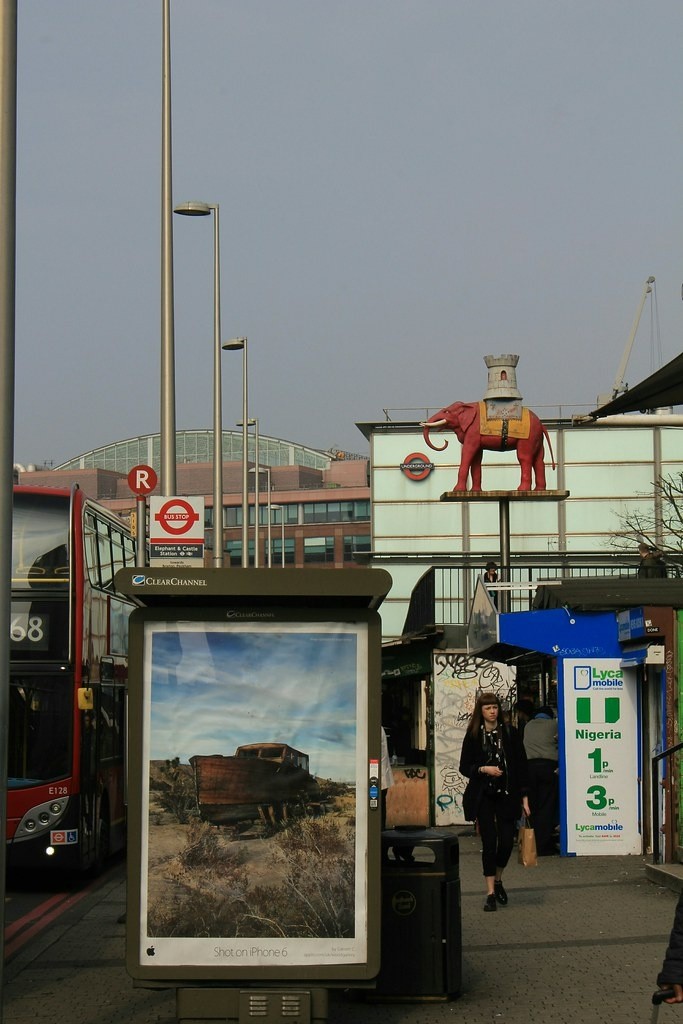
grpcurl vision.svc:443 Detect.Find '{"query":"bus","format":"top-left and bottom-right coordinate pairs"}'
top-left (2, 482), bottom-right (151, 894)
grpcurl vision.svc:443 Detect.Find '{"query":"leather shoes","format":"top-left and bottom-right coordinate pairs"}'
top-left (494, 880), bottom-right (507, 905)
top-left (484, 892), bottom-right (496, 911)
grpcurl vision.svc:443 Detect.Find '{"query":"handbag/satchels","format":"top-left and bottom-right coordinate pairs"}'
top-left (518, 815), bottom-right (537, 867)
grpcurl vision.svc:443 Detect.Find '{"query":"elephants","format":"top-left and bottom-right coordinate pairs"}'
top-left (419, 401), bottom-right (556, 491)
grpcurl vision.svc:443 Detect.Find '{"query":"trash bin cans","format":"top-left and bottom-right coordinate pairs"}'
top-left (380, 827), bottom-right (464, 1006)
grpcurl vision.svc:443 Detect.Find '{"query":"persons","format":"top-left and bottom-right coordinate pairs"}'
top-left (459, 693), bottom-right (530, 913)
top-left (523, 705), bottom-right (559, 856)
top-left (378, 725), bottom-right (393, 830)
top-left (657, 888), bottom-right (683, 1004)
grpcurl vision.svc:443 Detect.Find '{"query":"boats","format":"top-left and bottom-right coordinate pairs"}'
top-left (187, 741), bottom-right (323, 826)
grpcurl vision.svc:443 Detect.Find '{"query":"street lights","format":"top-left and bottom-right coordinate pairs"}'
top-left (221, 336), bottom-right (249, 571)
top-left (235, 417), bottom-right (260, 570)
top-left (249, 467), bottom-right (272, 569)
top-left (170, 200), bottom-right (224, 568)
top-left (266, 505), bottom-right (286, 569)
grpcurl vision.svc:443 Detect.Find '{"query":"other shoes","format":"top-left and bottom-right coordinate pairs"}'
top-left (539, 847), bottom-right (560, 856)
